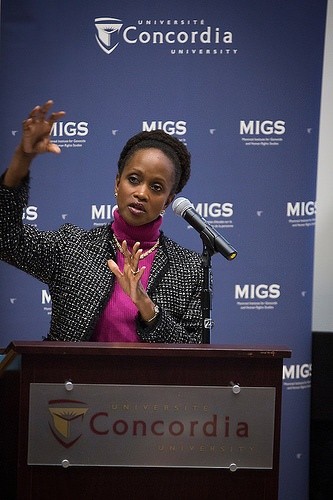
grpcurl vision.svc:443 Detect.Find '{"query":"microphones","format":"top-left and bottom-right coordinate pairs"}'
top-left (172, 197), bottom-right (237, 262)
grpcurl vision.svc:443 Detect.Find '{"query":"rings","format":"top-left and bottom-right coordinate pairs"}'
top-left (131, 267), bottom-right (140, 273)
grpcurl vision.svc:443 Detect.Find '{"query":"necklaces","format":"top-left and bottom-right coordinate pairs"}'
top-left (113, 233), bottom-right (160, 260)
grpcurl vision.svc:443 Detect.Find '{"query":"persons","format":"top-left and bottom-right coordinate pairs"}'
top-left (0, 100), bottom-right (215, 345)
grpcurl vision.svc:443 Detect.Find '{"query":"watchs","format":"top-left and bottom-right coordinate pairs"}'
top-left (145, 304), bottom-right (159, 323)
